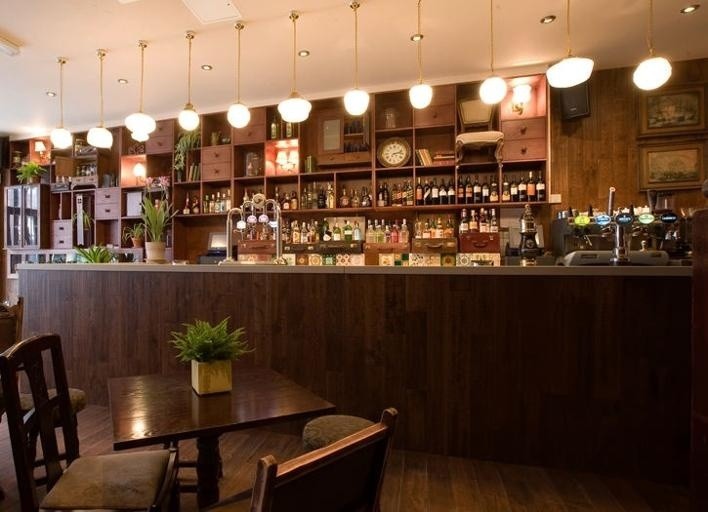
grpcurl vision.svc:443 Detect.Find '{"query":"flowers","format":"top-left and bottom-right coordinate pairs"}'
top-left (140, 177), bottom-right (179, 242)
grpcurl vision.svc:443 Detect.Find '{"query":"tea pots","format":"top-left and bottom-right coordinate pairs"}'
top-left (211, 131), bottom-right (222, 145)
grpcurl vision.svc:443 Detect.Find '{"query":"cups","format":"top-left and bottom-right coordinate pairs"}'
top-left (222, 137), bottom-right (231, 144)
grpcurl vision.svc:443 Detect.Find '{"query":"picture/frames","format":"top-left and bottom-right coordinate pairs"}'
top-left (637, 88), bottom-right (708, 138)
top-left (638, 143), bottom-right (706, 191)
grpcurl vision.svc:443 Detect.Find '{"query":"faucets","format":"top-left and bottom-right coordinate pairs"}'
top-left (226, 208), bottom-right (244, 259)
top-left (262, 199), bottom-right (281, 259)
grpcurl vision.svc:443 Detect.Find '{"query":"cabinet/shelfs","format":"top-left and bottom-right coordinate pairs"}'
top-left (3, 74), bottom-right (562, 267)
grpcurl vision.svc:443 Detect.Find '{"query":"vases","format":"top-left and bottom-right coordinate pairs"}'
top-left (143, 242), bottom-right (167, 264)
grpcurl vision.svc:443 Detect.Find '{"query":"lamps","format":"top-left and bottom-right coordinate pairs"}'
top-left (277, 10), bottom-right (312, 123)
top-left (409, 0), bottom-right (433, 110)
top-left (34, 141), bottom-right (46, 163)
top-left (50, 57), bottom-right (72, 149)
top-left (479, 0), bottom-right (507, 104)
top-left (227, 20), bottom-right (250, 128)
top-left (125, 40), bottom-right (156, 143)
top-left (86, 49), bottom-right (113, 149)
top-left (546, 0), bottom-right (594, 88)
top-left (178, 30), bottom-right (199, 131)
top-left (344, 1), bottom-right (369, 116)
top-left (632, 0), bottom-right (672, 90)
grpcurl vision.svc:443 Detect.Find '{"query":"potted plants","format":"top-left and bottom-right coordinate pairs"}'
top-left (122, 223), bottom-right (144, 247)
top-left (16, 160), bottom-right (47, 184)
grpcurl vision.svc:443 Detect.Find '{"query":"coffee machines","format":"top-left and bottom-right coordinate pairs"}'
top-left (518, 204), bottom-right (542, 268)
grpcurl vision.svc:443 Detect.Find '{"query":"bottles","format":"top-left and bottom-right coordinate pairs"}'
top-left (344, 142), bottom-right (367, 152)
top-left (75, 138), bottom-right (86, 156)
top-left (286, 119), bottom-right (295, 138)
top-left (345, 120), bottom-right (361, 134)
top-left (274, 170), bottom-right (546, 210)
top-left (76, 163), bottom-right (95, 177)
top-left (183, 188), bottom-right (264, 214)
top-left (12, 150), bottom-right (22, 168)
top-left (270, 115), bottom-right (279, 140)
top-left (250, 207), bottom-right (499, 243)
